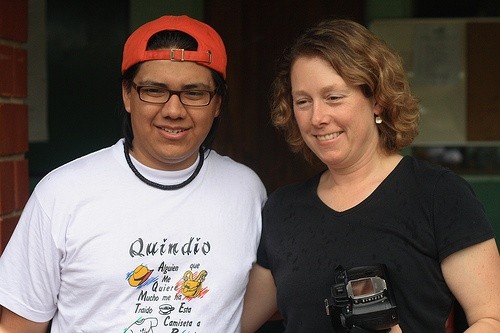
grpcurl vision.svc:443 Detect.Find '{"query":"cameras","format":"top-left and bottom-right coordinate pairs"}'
top-left (330, 264), bottom-right (399, 333)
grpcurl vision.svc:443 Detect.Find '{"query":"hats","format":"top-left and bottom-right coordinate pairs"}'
top-left (121, 16), bottom-right (227, 79)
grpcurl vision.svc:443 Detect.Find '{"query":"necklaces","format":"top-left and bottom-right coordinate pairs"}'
top-left (123, 137), bottom-right (205, 191)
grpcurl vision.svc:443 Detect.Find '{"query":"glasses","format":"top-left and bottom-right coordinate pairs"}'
top-left (130, 79), bottom-right (220, 107)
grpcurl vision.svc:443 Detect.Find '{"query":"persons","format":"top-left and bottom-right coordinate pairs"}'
top-left (240, 16), bottom-right (500, 333)
top-left (1, 13), bottom-right (270, 333)
